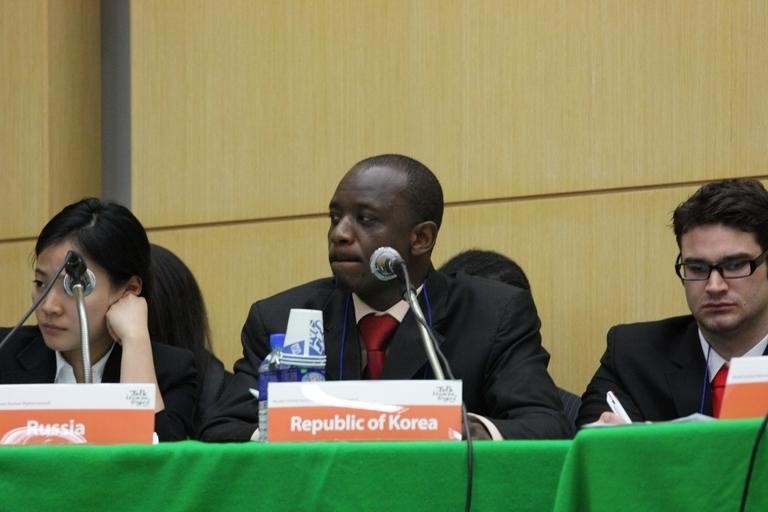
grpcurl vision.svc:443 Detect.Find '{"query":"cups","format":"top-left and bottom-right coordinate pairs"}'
top-left (278, 304), bottom-right (327, 381)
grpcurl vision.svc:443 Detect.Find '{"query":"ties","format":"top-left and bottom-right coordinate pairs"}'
top-left (354, 311), bottom-right (401, 380)
top-left (709, 365), bottom-right (730, 420)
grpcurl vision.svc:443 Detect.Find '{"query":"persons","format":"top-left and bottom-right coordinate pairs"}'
top-left (197, 151), bottom-right (570, 439)
top-left (0, 194), bottom-right (203, 440)
top-left (435, 246), bottom-right (583, 439)
top-left (145, 237), bottom-right (216, 351)
top-left (575, 177), bottom-right (768, 429)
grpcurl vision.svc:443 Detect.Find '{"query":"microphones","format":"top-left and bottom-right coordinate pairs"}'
top-left (64, 252), bottom-right (95, 298)
top-left (369, 247), bottom-right (407, 282)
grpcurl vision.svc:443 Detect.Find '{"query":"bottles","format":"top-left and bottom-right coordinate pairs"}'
top-left (256, 332), bottom-right (284, 442)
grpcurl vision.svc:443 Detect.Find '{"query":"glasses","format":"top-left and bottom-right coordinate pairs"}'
top-left (672, 247), bottom-right (766, 282)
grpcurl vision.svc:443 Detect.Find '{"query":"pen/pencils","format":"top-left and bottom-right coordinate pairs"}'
top-left (248, 387), bottom-right (259, 401)
top-left (605, 391), bottom-right (632, 424)
